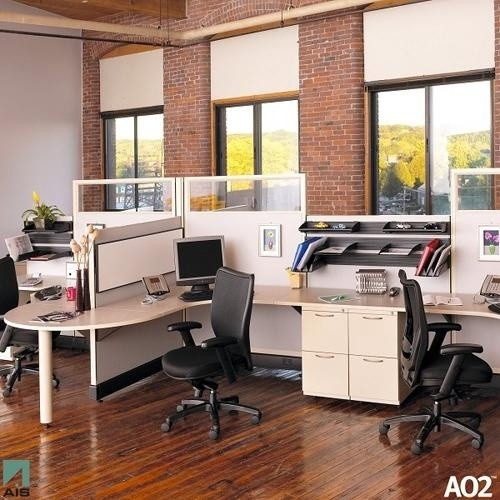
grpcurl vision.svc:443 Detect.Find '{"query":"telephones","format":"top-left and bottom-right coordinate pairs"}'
top-left (142, 275), bottom-right (170, 294)
top-left (480, 274), bottom-right (500, 297)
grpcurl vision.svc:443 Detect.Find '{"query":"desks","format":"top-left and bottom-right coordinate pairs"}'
top-left (4, 276), bottom-right (500, 430)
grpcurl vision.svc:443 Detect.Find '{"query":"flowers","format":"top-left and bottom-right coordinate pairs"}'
top-left (21, 190), bottom-right (66, 225)
top-left (268, 231), bottom-right (273, 238)
top-left (484, 232), bottom-right (499, 245)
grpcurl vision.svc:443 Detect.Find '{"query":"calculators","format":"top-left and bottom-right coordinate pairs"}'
top-left (20, 277), bottom-right (43, 287)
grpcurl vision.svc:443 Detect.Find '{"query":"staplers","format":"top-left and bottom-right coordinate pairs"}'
top-left (388, 287), bottom-right (401, 297)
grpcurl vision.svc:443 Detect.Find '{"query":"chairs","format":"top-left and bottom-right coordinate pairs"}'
top-left (380, 269), bottom-right (492, 456)
top-left (0, 255), bottom-right (66, 400)
top-left (159, 267), bottom-right (261, 443)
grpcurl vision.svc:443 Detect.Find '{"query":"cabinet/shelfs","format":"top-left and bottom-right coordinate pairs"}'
top-left (291, 221), bottom-right (452, 277)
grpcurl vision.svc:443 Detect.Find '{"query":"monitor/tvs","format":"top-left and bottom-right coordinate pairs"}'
top-left (173, 235), bottom-right (224, 292)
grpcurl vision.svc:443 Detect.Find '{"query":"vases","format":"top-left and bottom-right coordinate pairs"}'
top-left (82, 268), bottom-right (92, 311)
top-left (269, 239), bottom-right (273, 249)
top-left (31, 218), bottom-right (55, 231)
top-left (74, 269), bottom-right (84, 312)
top-left (489, 243), bottom-right (495, 255)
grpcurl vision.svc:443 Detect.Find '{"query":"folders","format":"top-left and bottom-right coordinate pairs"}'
top-left (292, 237), bottom-right (328, 272)
top-left (414, 239), bottom-right (442, 276)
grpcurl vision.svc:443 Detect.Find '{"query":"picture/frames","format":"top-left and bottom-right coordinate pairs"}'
top-left (86, 223), bottom-right (105, 230)
top-left (4, 233), bottom-right (34, 261)
top-left (259, 224), bottom-right (281, 258)
top-left (479, 226), bottom-right (499, 262)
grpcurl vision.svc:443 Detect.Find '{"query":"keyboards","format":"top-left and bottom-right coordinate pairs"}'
top-left (181, 293), bottom-right (212, 302)
top-left (35, 286), bottom-right (62, 301)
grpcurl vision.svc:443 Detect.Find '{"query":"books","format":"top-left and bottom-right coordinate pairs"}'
top-left (422, 295), bottom-right (463, 306)
top-left (31, 310), bottom-right (77, 325)
top-left (29, 254), bottom-right (57, 260)
top-left (319, 293), bottom-right (359, 303)
top-left (380, 246), bottom-right (411, 255)
top-left (291, 236), bottom-right (347, 271)
